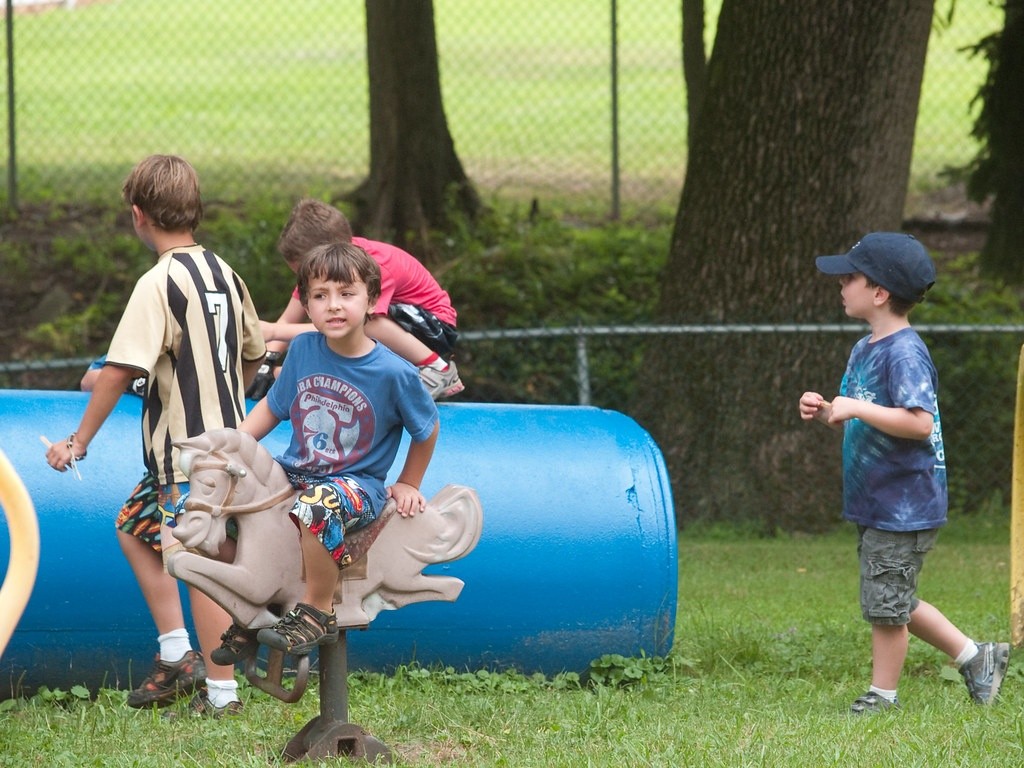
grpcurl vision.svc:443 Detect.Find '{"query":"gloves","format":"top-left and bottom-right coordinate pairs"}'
top-left (245, 350), bottom-right (282, 400)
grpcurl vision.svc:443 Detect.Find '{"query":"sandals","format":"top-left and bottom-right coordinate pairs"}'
top-left (210, 604), bottom-right (280, 666)
top-left (256, 602), bottom-right (339, 654)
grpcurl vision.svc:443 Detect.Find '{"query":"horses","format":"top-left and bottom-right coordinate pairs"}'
top-left (167, 426), bottom-right (482, 632)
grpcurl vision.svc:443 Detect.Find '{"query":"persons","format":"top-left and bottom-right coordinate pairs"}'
top-left (196, 240), bottom-right (440, 665)
top-left (259, 200), bottom-right (466, 403)
top-left (45, 155), bottom-right (266, 717)
top-left (799, 231), bottom-right (1010, 716)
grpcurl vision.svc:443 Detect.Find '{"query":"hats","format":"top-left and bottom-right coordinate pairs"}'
top-left (816, 232), bottom-right (936, 304)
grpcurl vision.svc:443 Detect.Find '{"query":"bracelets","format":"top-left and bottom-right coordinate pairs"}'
top-left (67, 433), bottom-right (88, 482)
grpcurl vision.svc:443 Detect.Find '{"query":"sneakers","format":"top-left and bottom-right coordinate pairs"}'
top-left (126, 650), bottom-right (207, 709)
top-left (159, 689), bottom-right (242, 720)
top-left (958, 642), bottom-right (1010, 706)
top-left (850, 691), bottom-right (897, 714)
top-left (415, 354), bottom-right (466, 401)
